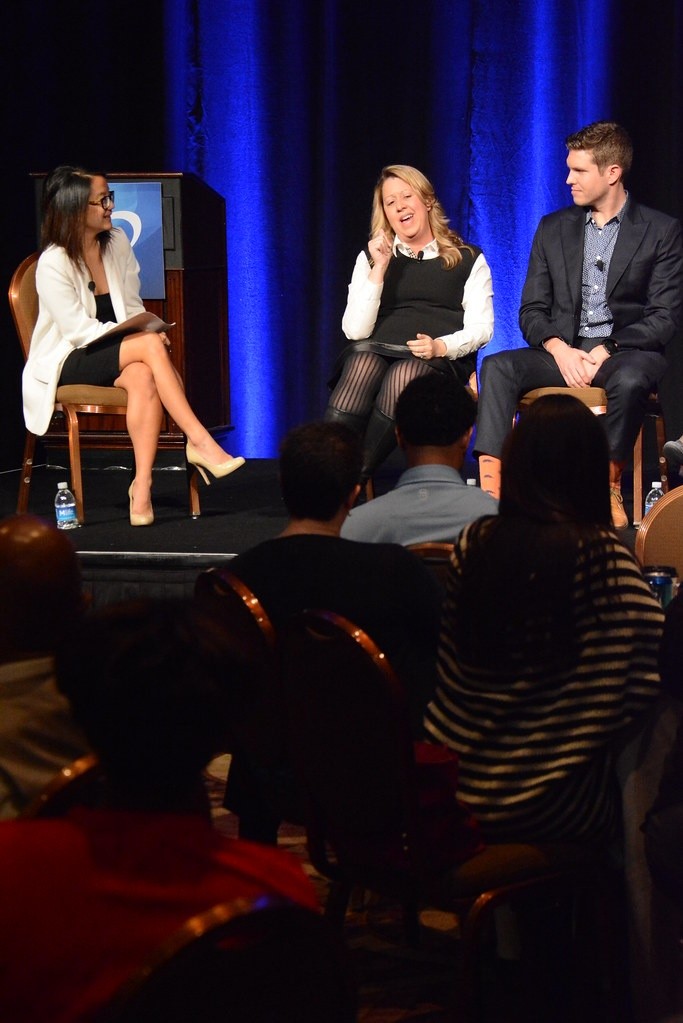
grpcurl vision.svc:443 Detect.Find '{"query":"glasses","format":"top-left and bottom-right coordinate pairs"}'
top-left (87, 190), bottom-right (115, 209)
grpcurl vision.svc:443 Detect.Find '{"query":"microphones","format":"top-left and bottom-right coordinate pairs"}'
top-left (88, 282), bottom-right (96, 290)
top-left (418, 250), bottom-right (423, 262)
top-left (597, 260), bottom-right (603, 271)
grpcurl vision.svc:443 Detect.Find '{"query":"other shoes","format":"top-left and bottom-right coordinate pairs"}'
top-left (609, 484), bottom-right (629, 529)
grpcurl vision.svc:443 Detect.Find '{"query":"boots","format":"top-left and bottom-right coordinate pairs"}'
top-left (359, 402), bottom-right (398, 475)
top-left (322, 405), bottom-right (369, 440)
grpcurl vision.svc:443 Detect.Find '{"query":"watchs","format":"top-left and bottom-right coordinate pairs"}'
top-left (603, 341), bottom-right (618, 355)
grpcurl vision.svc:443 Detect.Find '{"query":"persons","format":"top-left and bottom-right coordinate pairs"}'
top-left (474, 120), bottom-right (683, 527)
top-left (0, 512), bottom-right (102, 820)
top-left (327, 165), bottom-right (495, 488)
top-left (340, 373), bottom-right (500, 547)
top-left (421, 393), bottom-right (665, 849)
top-left (22, 164), bottom-right (245, 526)
top-left (640, 588), bottom-right (683, 911)
top-left (224, 419), bottom-right (460, 966)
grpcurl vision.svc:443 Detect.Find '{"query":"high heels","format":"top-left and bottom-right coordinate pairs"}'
top-left (128, 479), bottom-right (154, 526)
top-left (186, 443), bottom-right (245, 485)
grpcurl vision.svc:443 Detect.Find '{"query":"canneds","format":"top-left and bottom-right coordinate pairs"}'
top-left (643, 572), bottom-right (672, 607)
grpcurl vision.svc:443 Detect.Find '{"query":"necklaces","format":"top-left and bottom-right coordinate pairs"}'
top-left (404, 244), bottom-right (416, 258)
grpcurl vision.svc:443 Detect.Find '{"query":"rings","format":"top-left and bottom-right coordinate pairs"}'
top-left (420, 356), bottom-right (423, 358)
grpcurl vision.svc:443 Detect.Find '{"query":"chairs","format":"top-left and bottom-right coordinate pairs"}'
top-left (7, 246), bottom-right (204, 521)
top-left (512, 377), bottom-right (668, 532)
top-left (364, 370), bottom-right (479, 504)
top-left (92, 540), bottom-right (612, 1022)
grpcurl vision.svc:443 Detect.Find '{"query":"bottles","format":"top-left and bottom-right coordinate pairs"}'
top-left (56, 482), bottom-right (78, 531)
top-left (645, 482), bottom-right (664, 515)
